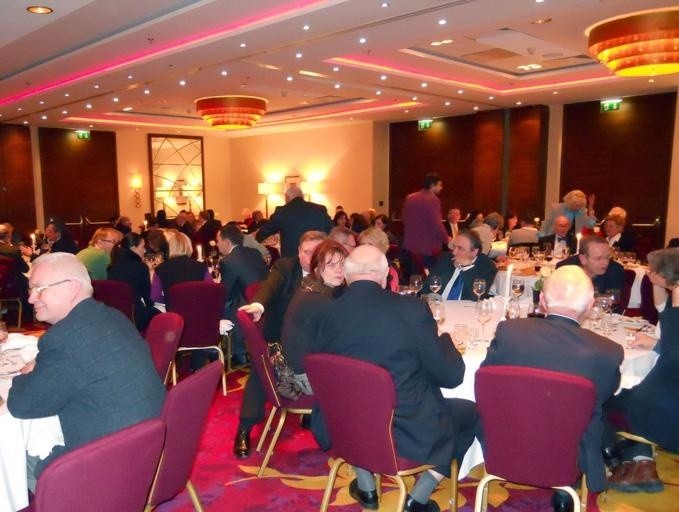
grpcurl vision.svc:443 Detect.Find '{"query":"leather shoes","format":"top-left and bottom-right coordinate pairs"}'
top-left (403, 494), bottom-right (440, 512)
top-left (603, 443), bottom-right (622, 469)
top-left (231, 354), bottom-right (250, 365)
top-left (233, 429), bottom-right (251, 460)
top-left (606, 458), bottom-right (665, 493)
top-left (550, 490), bottom-right (572, 512)
top-left (347, 477), bottom-right (379, 510)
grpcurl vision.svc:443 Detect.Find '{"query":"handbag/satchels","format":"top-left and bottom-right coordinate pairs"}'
top-left (274, 351), bottom-right (303, 401)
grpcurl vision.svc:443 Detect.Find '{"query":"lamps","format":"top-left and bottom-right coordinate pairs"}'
top-left (129, 173), bottom-right (142, 208)
top-left (196, 97), bottom-right (267, 130)
top-left (257, 182), bottom-right (272, 219)
top-left (587, 10), bottom-right (679, 77)
top-left (27, 6), bottom-right (53, 14)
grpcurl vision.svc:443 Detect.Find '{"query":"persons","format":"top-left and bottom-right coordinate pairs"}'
top-left (502, 208), bottom-right (522, 238)
top-left (329, 203), bottom-right (406, 254)
top-left (0, 221), bottom-right (34, 330)
top-left (541, 189), bottom-right (596, 238)
top-left (602, 216), bottom-right (640, 256)
top-left (19, 220), bottom-right (80, 266)
top-left (216, 223), bottom-right (272, 367)
top-left (254, 182), bottom-right (335, 257)
top-left (477, 265), bottom-right (632, 511)
top-left (281, 239), bottom-right (350, 453)
top-left (469, 212), bottom-right (505, 259)
top-left (396, 172), bottom-right (451, 285)
top-left (315, 243), bottom-right (474, 508)
top-left (417, 229), bottom-right (502, 302)
top-left (150, 231), bottom-right (214, 303)
top-left (555, 236), bottom-right (624, 315)
top-left (539, 218), bottom-right (577, 260)
top-left (75, 227), bottom-right (124, 281)
top-left (601, 246), bottom-right (679, 494)
top-left (442, 204), bottom-right (469, 241)
top-left (232, 230), bottom-right (330, 464)
top-left (7, 252), bottom-right (167, 497)
top-left (111, 204), bottom-right (269, 246)
top-left (106, 232), bottom-right (164, 335)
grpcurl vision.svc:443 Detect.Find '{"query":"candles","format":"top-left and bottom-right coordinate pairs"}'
top-left (197, 249), bottom-right (202, 259)
top-left (576, 238), bottom-right (580, 254)
top-left (33, 240), bottom-right (35, 252)
top-left (145, 224), bottom-right (147, 231)
top-left (505, 271), bottom-right (511, 297)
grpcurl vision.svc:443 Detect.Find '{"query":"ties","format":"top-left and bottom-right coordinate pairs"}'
top-left (446, 269), bottom-right (464, 300)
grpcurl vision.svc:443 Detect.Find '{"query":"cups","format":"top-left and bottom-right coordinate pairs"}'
top-left (590, 307), bottom-right (601, 322)
top-left (623, 257), bottom-right (628, 265)
top-left (512, 279), bottom-right (525, 301)
top-left (505, 303), bottom-right (520, 320)
top-left (508, 246), bottom-right (529, 258)
top-left (532, 247), bottom-right (539, 258)
top-left (545, 250), bottom-right (554, 262)
top-left (473, 277), bottom-right (486, 301)
top-left (475, 302), bottom-right (493, 343)
top-left (453, 324), bottom-right (467, 354)
top-left (607, 289), bottom-right (621, 313)
top-left (562, 247), bottom-right (569, 259)
top-left (533, 262), bottom-right (541, 272)
top-left (0, 322), bottom-right (8, 346)
top-left (409, 274), bottom-right (423, 296)
top-left (432, 303), bottom-right (446, 324)
top-left (429, 275), bottom-right (442, 293)
top-left (528, 302), bottom-right (545, 318)
top-left (601, 320), bottom-right (613, 337)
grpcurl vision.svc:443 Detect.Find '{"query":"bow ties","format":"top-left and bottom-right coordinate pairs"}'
top-left (557, 236), bottom-right (568, 242)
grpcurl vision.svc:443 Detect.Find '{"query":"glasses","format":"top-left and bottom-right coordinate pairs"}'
top-left (101, 238), bottom-right (117, 244)
top-left (27, 279), bottom-right (71, 296)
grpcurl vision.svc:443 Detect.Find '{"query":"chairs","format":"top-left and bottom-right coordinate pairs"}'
top-left (168, 281), bottom-right (227, 396)
top-left (244, 282), bottom-right (265, 324)
top-left (146, 313), bottom-right (184, 385)
top-left (262, 244), bottom-right (280, 265)
top-left (236, 309), bottom-right (316, 479)
top-left (474, 365), bottom-right (596, 512)
top-left (640, 274), bottom-right (659, 326)
top-left (91, 280), bottom-right (136, 326)
top-left (633, 235), bottom-right (652, 261)
top-left (303, 353), bottom-right (458, 511)
top-left (0, 256), bottom-right (23, 329)
top-left (622, 268), bottom-right (636, 316)
top-left (146, 360), bottom-right (223, 511)
top-left (507, 242), bottom-right (539, 258)
top-left (244, 218), bottom-right (255, 228)
top-left (386, 244), bottom-right (424, 277)
top-left (34, 417), bottom-right (167, 512)
